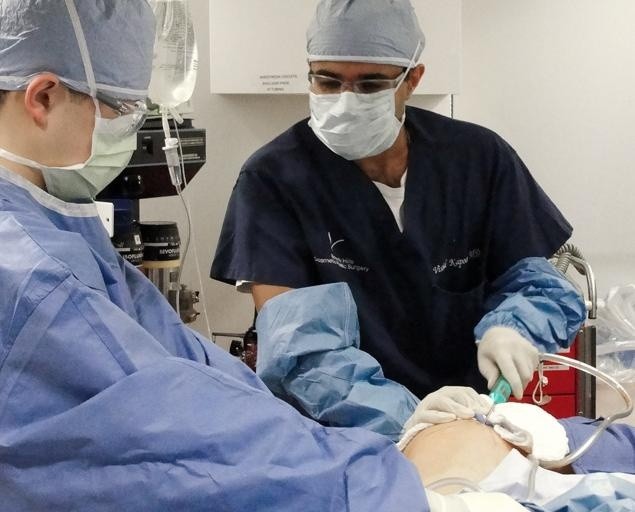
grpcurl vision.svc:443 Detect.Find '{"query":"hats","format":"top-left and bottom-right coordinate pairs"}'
top-left (1, 0), bottom-right (156, 99)
top-left (306, 0), bottom-right (425, 66)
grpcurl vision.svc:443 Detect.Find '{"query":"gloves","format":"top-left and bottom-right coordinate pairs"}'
top-left (477, 327), bottom-right (539, 399)
top-left (434, 491), bottom-right (530, 512)
top-left (402, 385), bottom-right (493, 428)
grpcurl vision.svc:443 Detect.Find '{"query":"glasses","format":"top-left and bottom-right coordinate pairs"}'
top-left (16, 71), bottom-right (147, 138)
top-left (308, 67), bottom-right (407, 95)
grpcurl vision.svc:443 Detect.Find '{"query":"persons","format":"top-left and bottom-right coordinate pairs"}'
top-left (2, 1), bottom-right (634, 511)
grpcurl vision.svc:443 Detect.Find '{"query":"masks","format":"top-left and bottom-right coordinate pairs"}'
top-left (308, 63), bottom-right (411, 162)
top-left (40, 116), bottom-right (137, 203)
